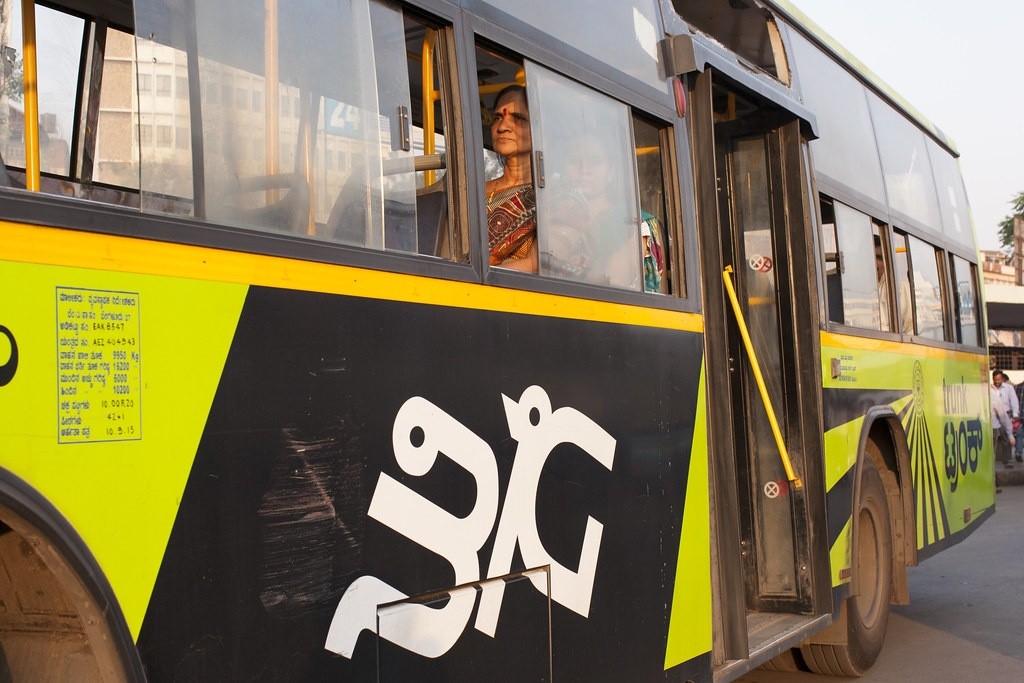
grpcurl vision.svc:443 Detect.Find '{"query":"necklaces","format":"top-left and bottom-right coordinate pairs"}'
top-left (489, 178), bottom-right (499, 204)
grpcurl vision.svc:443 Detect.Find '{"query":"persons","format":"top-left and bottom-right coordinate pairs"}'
top-left (874, 234), bottom-right (890, 331)
top-left (641, 210), bottom-right (664, 291)
top-left (485, 85), bottom-right (538, 274)
top-left (992, 371), bottom-right (1024, 493)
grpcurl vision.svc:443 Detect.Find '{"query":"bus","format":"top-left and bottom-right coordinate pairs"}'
top-left (1, 0), bottom-right (996, 682)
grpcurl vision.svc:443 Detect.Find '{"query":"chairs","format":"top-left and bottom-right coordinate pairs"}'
top-left (187, 151), bottom-right (980, 357)
